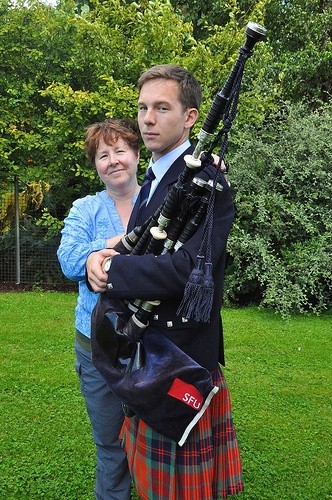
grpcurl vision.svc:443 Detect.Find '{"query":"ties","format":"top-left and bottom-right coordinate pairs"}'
top-left (138, 166), bottom-right (156, 212)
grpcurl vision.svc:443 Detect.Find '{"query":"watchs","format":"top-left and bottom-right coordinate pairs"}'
top-left (104, 258), bottom-right (111, 276)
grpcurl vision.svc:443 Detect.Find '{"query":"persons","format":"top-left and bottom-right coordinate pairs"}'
top-left (85, 64), bottom-right (244, 500)
top-left (57, 119), bottom-right (142, 500)
